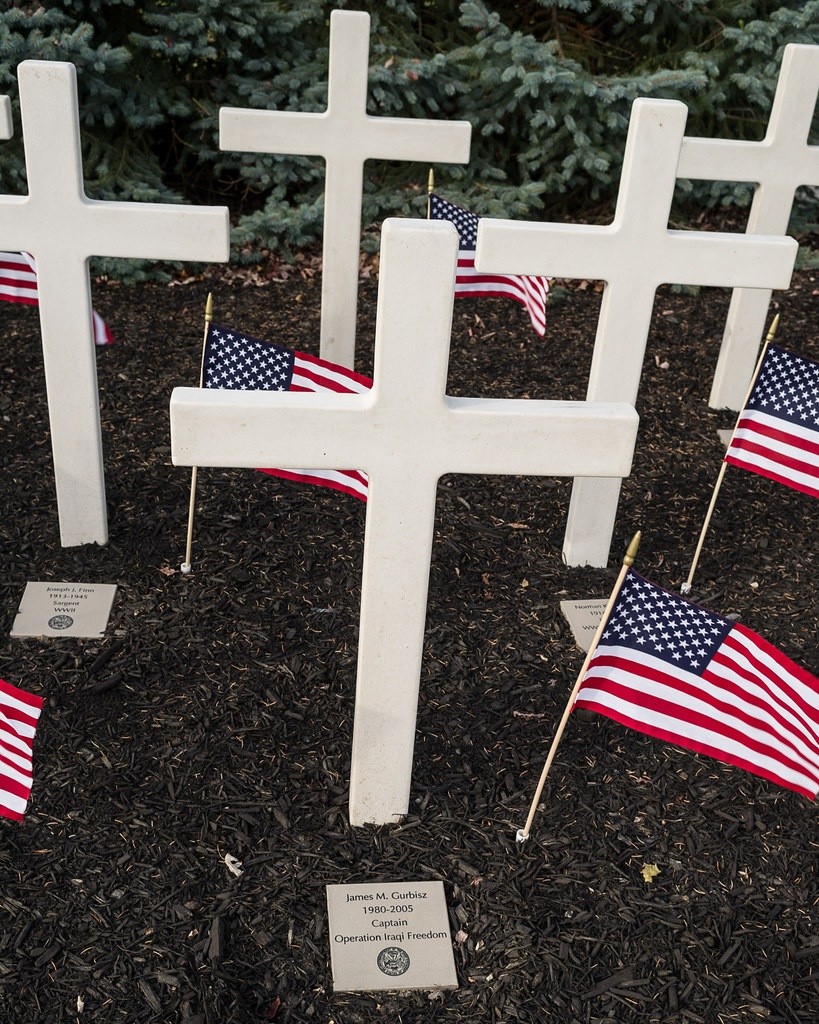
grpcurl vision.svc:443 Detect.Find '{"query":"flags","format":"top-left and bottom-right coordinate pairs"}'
top-left (1, 250), bottom-right (116, 345)
top-left (200, 325), bottom-right (374, 502)
top-left (567, 569), bottom-right (819, 803)
top-left (723, 347), bottom-right (819, 501)
top-left (0, 680), bottom-right (44, 825)
top-left (422, 194), bottom-right (553, 341)
